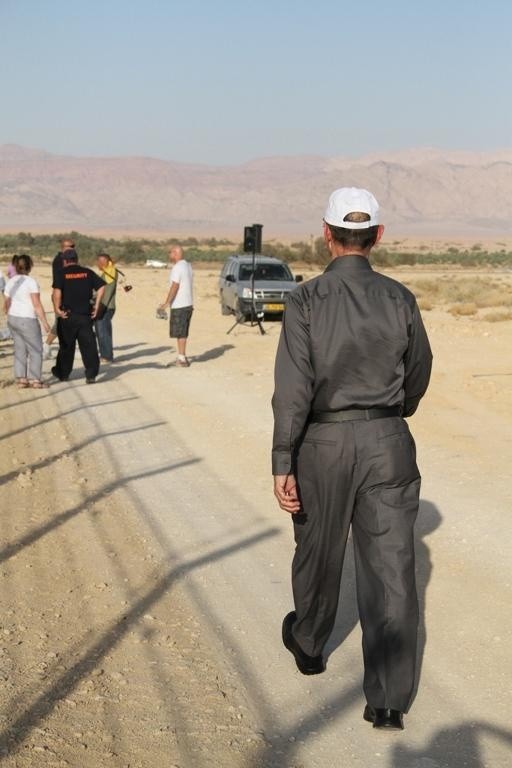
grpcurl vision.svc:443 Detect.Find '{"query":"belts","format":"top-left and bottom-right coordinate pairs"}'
top-left (309, 404), bottom-right (405, 424)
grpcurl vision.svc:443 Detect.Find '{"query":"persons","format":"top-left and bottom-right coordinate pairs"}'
top-left (49, 247), bottom-right (107, 385)
top-left (0, 254), bottom-right (51, 389)
top-left (269, 183), bottom-right (436, 732)
top-left (93, 253), bottom-right (121, 364)
top-left (158, 244), bottom-right (195, 370)
top-left (41, 238), bottom-right (78, 358)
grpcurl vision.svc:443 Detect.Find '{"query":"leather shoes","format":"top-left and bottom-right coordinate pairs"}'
top-left (363, 700), bottom-right (404, 731)
top-left (282, 611), bottom-right (325, 675)
top-left (51, 366), bottom-right (68, 381)
top-left (86, 378), bottom-right (96, 384)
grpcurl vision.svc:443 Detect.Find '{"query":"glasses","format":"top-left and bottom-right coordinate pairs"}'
top-left (66, 245), bottom-right (75, 248)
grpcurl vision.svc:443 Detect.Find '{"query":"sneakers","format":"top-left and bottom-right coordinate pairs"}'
top-left (99, 357), bottom-right (112, 366)
top-left (166, 356), bottom-right (190, 367)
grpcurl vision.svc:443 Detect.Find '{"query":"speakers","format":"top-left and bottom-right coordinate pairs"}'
top-left (244, 225), bottom-right (262, 252)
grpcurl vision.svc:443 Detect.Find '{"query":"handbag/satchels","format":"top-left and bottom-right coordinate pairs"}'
top-left (90, 297), bottom-right (108, 320)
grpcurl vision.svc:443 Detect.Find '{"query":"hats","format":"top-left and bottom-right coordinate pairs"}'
top-left (324, 186), bottom-right (381, 230)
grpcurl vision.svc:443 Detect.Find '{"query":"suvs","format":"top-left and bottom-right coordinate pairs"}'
top-left (146, 259), bottom-right (168, 268)
top-left (218, 252), bottom-right (303, 323)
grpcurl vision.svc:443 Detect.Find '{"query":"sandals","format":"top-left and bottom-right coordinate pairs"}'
top-left (32, 381), bottom-right (49, 388)
top-left (18, 382), bottom-right (29, 388)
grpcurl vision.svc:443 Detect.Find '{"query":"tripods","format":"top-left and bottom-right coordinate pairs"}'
top-left (226, 261), bottom-right (269, 337)
